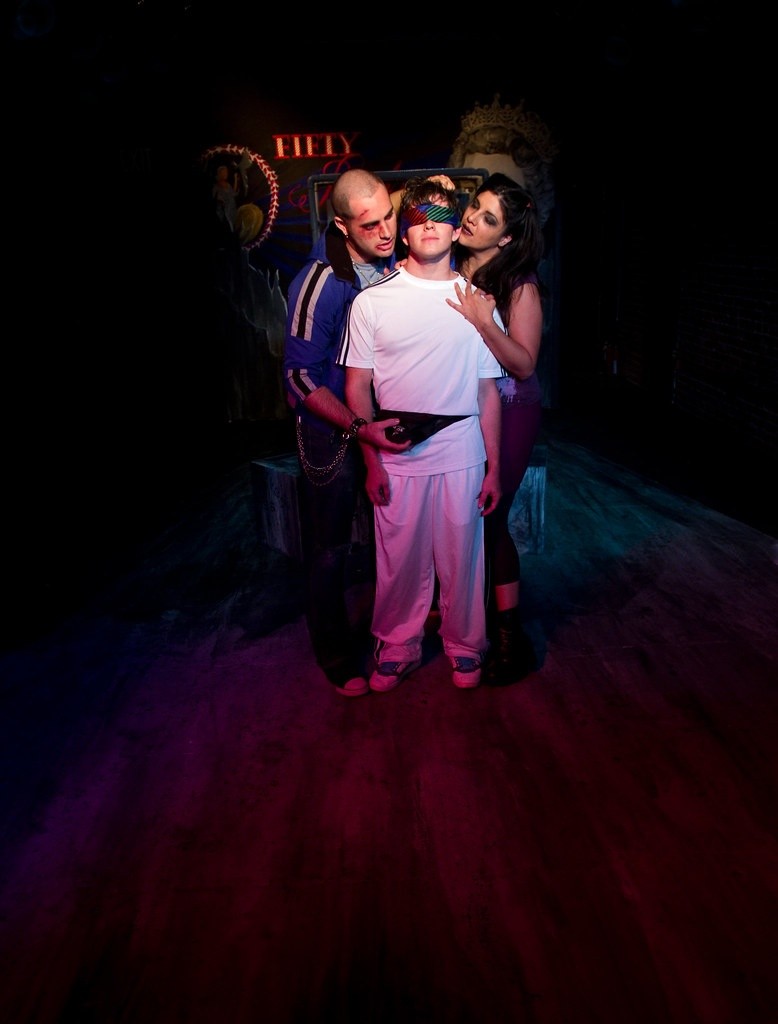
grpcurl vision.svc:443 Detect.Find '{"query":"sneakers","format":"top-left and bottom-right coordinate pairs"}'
top-left (331, 676), bottom-right (368, 695)
top-left (447, 653), bottom-right (483, 688)
top-left (371, 659), bottom-right (423, 691)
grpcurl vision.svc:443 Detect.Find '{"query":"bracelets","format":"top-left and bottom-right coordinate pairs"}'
top-left (344, 418), bottom-right (366, 438)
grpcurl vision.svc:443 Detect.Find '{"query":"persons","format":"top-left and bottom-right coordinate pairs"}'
top-left (337, 179), bottom-right (514, 689)
top-left (392, 173), bottom-right (544, 682)
top-left (277, 167), bottom-right (407, 696)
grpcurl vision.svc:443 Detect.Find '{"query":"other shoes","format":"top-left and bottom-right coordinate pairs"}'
top-left (489, 649), bottom-right (543, 685)
